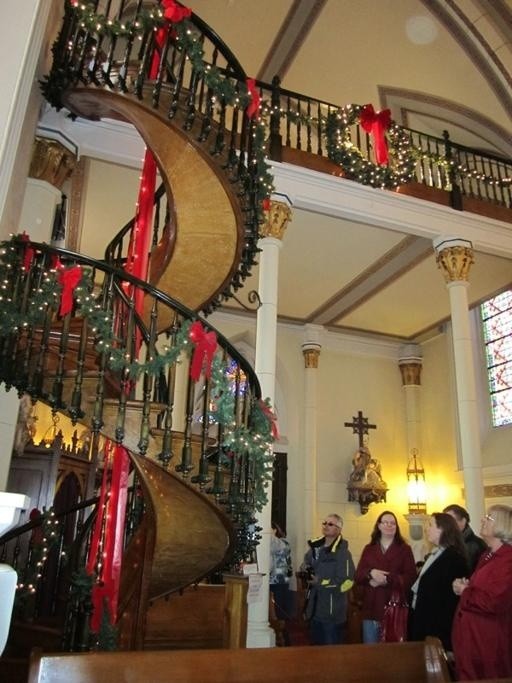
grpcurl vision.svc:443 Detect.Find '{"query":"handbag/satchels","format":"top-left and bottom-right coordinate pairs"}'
top-left (380, 598), bottom-right (409, 643)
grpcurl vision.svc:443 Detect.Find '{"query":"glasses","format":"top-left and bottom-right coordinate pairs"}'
top-left (323, 521), bottom-right (340, 528)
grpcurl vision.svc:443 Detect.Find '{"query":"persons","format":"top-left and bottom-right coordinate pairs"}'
top-left (404, 512), bottom-right (467, 647)
top-left (301, 512), bottom-right (355, 647)
top-left (448, 504), bottom-right (511, 682)
top-left (443, 504), bottom-right (485, 565)
top-left (271, 525), bottom-right (294, 620)
top-left (351, 510), bottom-right (417, 646)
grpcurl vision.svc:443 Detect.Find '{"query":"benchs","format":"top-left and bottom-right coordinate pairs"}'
top-left (28, 635), bottom-right (456, 683)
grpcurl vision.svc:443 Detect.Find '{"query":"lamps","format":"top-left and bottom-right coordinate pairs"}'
top-left (405, 447), bottom-right (431, 515)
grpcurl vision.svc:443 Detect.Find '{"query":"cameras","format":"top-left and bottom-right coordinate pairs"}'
top-left (298, 567), bottom-right (314, 580)
